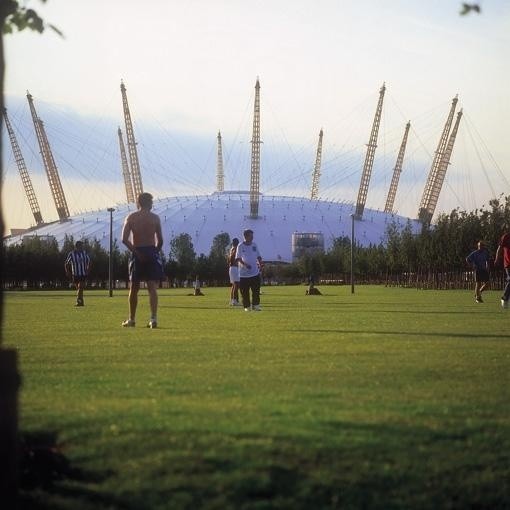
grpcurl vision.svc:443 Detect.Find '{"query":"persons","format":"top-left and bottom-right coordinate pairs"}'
top-left (466, 240), bottom-right (491, 304)
top-left (64, 241), bottom-right (92, 306)
top-left (234, 229), bottom-right (265, 311)
top-left (227, 237), bottom-right (241, 306)
top-left (493, 231), bottom-right (510, 308)
top-left (121, 193), bottom-right (165, 329)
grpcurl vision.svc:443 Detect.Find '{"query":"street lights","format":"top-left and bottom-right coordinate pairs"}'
top-left (107, 208), bottom-right (115, 297)
top-left (349, 214), bottom-right (356, 293)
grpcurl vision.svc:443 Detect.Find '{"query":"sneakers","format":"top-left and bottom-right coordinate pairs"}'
top-left (229, 299), bottom-right (263, 312)
top-left (121, 320), bottom-right (136, 328)
top-left (146, 321), bottom-right (159, 329)
